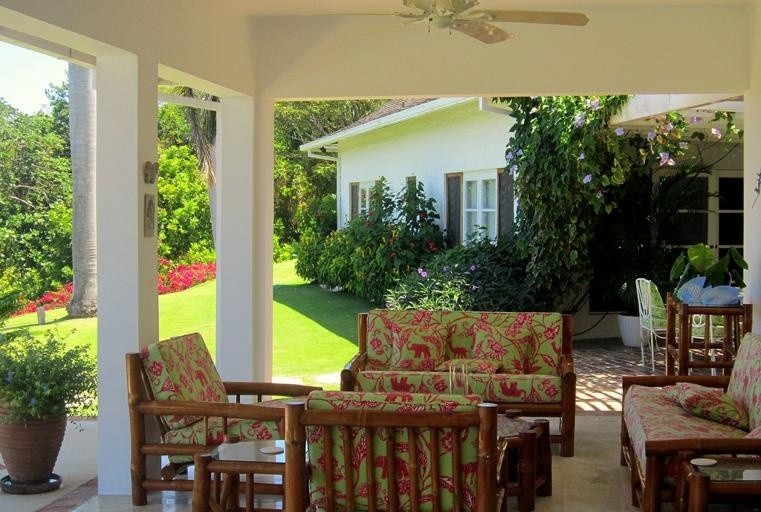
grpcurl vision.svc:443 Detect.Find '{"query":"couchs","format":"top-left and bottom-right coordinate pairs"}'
top-left (619, 332), bottom-right (761, 512)
top-left (340, 307), bottom-right (577, 457)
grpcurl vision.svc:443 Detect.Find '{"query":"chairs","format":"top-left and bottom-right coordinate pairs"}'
top-left (284, 389), bottom-right (509, 512)
top-left (126, 334), bottom-right (323, 506)
top-left (634, 277), bottom-right (742, 376)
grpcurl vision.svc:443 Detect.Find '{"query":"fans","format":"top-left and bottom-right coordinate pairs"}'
top-left (303, 0), bottom-right (590, 44)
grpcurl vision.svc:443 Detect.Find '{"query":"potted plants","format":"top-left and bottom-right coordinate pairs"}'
top-left (612, 270), bottom-right (674, 348)
top-left (0, 325), bottom-right (100, 483)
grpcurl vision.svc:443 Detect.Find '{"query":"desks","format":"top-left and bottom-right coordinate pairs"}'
top-left (497, 409), bottom-right (552, 512)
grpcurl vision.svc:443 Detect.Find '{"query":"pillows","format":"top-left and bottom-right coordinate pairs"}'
top-left (661, 380), bottom-right (749, 430)
top-left (391, 323), bottom-right (534, 375)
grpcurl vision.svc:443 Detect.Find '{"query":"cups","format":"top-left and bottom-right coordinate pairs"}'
top-left (449, 363), bottom-right (468, 396)
